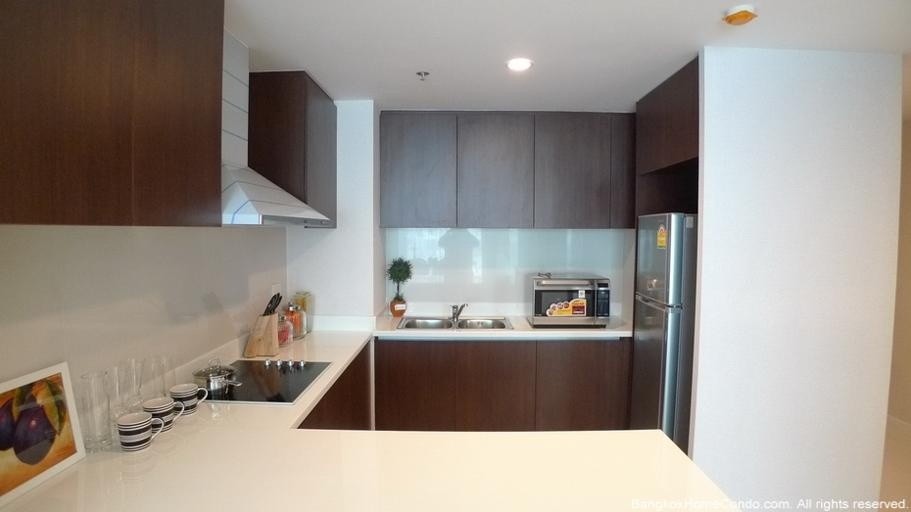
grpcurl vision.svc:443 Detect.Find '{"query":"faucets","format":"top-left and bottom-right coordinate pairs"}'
top-left (452, 303), bottom-right (468, 322)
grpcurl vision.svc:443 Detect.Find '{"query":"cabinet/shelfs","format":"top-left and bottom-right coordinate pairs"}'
top-left (455, 112), bottom-right (537, 229)
top-left (454, 340), bottom-right (534, 431)
top-left (613, 115), bottom-right (637, 225)
top-left (379, 113), bottom-right (458, 229)
top-left (378, 341), bottom-right (455, 431)
top-left (249, 72), bottom-right (338, 226)
top-left (2, 2), bottom-right (224, 228)
top-left (632, 55), bottom-right (698, 176)
top-left (535, 113), bottom-right (612, 228)
top-left (537, 340), bottom-right (631, 426)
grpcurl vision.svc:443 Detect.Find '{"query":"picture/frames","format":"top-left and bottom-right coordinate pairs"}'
top-left (2, 359), bottom-right (87, 504)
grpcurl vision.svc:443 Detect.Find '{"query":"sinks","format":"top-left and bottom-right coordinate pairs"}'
top-left (458, 318), bottom-right (506, 329)
top-left (405, 318), bottom-right (452, 330)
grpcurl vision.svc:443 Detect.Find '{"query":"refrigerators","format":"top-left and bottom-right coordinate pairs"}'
top-left (626, 211), bottom-right (695, 454)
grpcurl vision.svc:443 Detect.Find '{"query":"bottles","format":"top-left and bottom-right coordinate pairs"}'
top-left (278, 301), bottom-right (308, 347)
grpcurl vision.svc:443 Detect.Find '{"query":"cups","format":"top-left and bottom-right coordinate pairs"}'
top-left (142, 396), bottom-right (185, 433)
top-left (118, 410), bottom-right (165, 453)
top-left (169, 383), bottom-right (209, 415)
top-left (79, 349), bottom-right (176, 454)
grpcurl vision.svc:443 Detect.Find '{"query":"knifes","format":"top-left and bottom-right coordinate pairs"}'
top-left (261, 290), bottom-right (284, 316)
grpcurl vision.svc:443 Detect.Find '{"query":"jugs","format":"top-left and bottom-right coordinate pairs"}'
top-left (198, 358), bottom-right (335, 404)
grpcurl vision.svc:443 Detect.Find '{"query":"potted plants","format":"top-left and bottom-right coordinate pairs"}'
top-left (388, 257), bottom-right (412, 317)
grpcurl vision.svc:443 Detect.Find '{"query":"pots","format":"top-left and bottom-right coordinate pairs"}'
top-left (192, 356), bottom-right (244, 396)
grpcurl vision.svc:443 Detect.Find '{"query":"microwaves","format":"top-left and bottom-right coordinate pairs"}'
top-left (524, 271), bottom-right (612, 328)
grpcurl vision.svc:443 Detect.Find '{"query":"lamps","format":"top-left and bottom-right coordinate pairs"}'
top-left (721, 6), bottom-right (756, 25)
